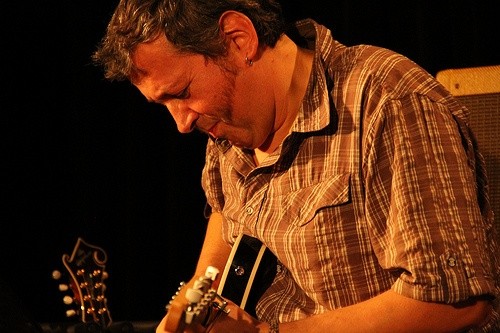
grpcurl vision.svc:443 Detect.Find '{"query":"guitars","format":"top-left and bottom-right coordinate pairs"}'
top-left (166, 266), bottom-right (230, 332)
top-left (51, 237), bottom-right (113, 333)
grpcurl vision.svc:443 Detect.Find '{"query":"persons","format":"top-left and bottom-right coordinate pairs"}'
top-left (91, 0), bottom-right (500, 333)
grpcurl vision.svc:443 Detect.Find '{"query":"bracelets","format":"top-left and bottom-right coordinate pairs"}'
top-left (268, 319), bottom-right (279, 333)
